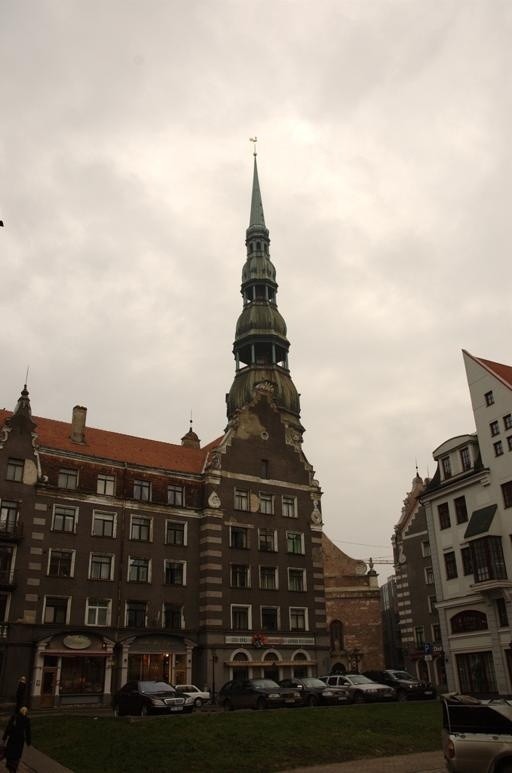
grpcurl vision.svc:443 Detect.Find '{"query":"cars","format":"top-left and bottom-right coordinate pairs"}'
top-left (280, 677), bottom-right (351, 707)
top-left (319, 673), bottom-right (395, 703)
top-left (364, 669), bottom-right (435, 701)
top-left (174, 683), bottom-right (211, 708)
top-left (111, 679), bottom-right (194, 716)
top-left (218, 677), bottom-right (303, 710)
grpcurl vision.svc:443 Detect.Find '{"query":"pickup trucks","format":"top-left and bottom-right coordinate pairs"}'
top-left (442, 694), bottom-right (511, 771)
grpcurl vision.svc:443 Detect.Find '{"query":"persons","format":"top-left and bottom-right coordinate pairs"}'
top-left (1, 705), bottom-right (33, 772)
top-left (16, 675), bottom-right (27, 710)
